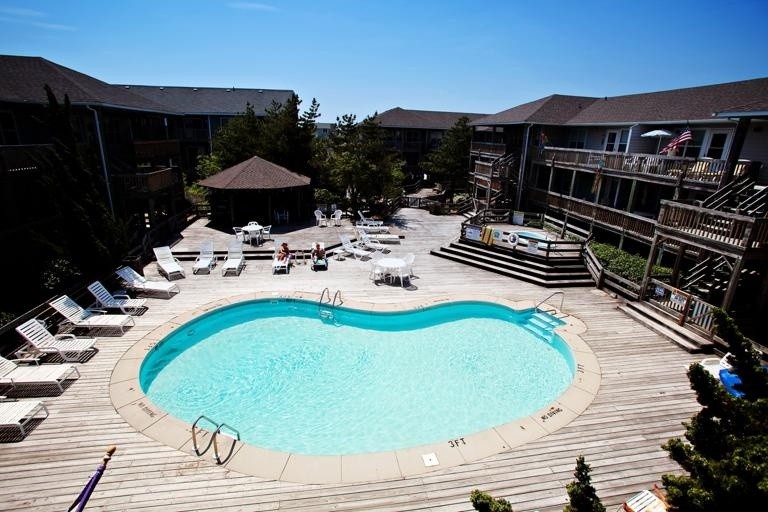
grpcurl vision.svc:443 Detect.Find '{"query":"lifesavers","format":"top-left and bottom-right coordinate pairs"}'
top-left (507, 233), bottom-right (519, 247)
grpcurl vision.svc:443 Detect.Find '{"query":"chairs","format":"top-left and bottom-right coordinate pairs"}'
top-left (699, 346), bottom-right (763, 385)
top-left (667, 156), bottom-right (751, 183)
top-left (271, 210), bottom-right (399, 275)
top-left (15, 318), bottom-right (99, 364)
top-left (88, 280), bottom-right (148, 316)
top-left (0, 356), bottom-right (81, 396)
top-left (221, 242), bottom-right (246, 276)
top-left (115, 266), bottom-right (181, 298)
top-left (1, 395), bottom-right (49, 436)
top-left (616, 484), bottom-right (671, 512)
top-left (192, 240), bottom-right (217, 275)
top-left (369, 250), bottom-right (416, 288)
top-left (153, 246), bottom-right (186, 281)
top-left (232, 221), bottom-right (272, 247)
top-left (49, 295), bottom-right (136, 337)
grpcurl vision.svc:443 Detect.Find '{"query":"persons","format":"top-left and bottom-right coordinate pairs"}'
top-left (277, 243), bottom-right (292, 261)
top-left (313, 243), bottom-right (323, 264)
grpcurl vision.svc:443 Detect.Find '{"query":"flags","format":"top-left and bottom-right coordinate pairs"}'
top-left (659, 128), bottom-right (693, 154)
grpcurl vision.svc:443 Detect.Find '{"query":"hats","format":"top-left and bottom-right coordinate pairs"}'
top-left (282, 243), bottom-right (287, 246)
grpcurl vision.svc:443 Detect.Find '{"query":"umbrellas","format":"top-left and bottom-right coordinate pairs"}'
top-left (640, 130), bottom-right (672, 155)
